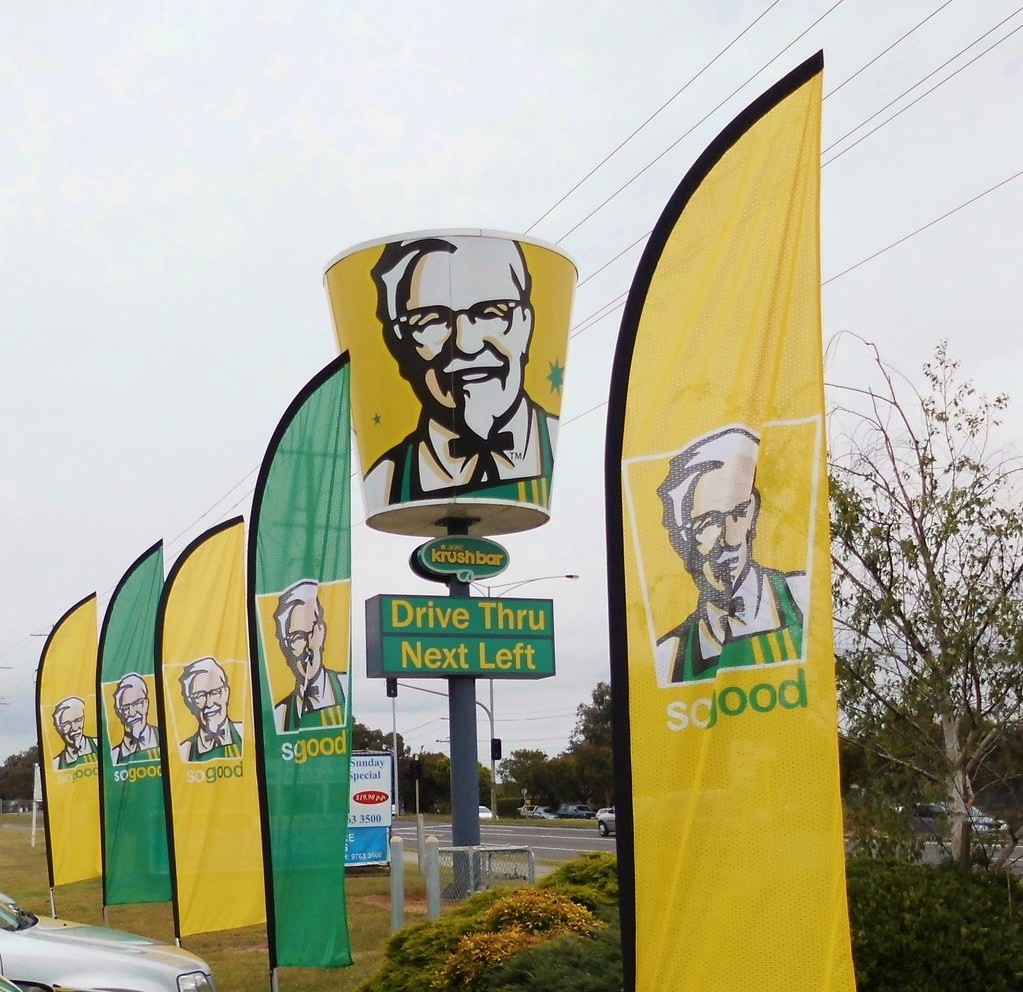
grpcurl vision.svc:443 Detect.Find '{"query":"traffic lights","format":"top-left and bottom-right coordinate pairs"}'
top-left (491, 739), bottom-right (502, 761)
top-left (387, 678), bottom-right (398, 697)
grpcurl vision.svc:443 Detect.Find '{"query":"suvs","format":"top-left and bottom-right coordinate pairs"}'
top-left (557, 805), bottom-right (596, 820)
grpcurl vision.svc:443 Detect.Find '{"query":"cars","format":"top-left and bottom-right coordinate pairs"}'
top-left (596, 808), bottom-right (612, 819)
top-left (0, 892), bottom-right (218, 992)
top-left (898, 801), bottom-right (1009, 835)
top-left (531, 806), bottom-right (558, 819)
top-left (479, 806), bottom-right (499, 821)
top-left (598, 806), bottom-right (616, 837)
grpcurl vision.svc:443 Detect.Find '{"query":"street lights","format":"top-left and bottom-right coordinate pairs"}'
top-left (470, 574), bottom-right (581, 820)
